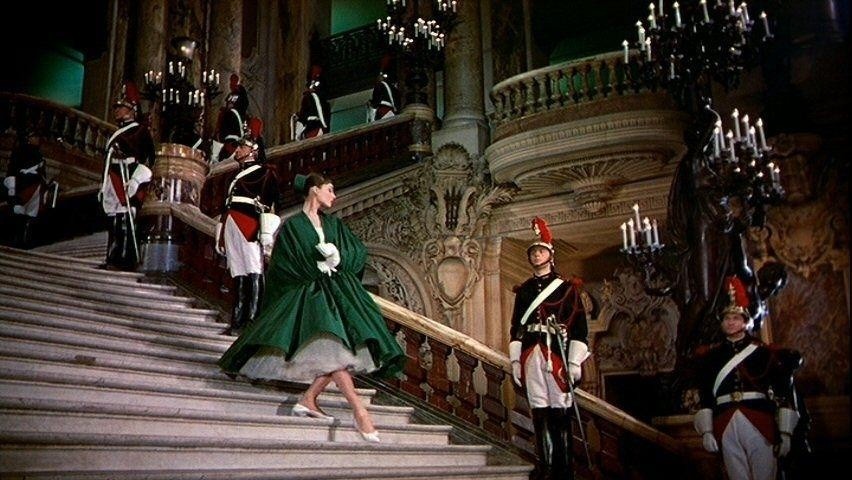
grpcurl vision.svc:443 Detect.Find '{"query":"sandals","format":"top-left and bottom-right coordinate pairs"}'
top-left (350, 418), bottom-right (382, 445)
top-left (290, 403), bottom-right (336, 420)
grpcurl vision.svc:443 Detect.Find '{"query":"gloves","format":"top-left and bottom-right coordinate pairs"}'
top-left (262, 243), bottom-right (273, 262)
top-left (702, 431), bottom-right (720, 453)
top-left (315, 259), bottom-right (338, 276)
top-left (776, 434), bottom-right (791, 458)
top-left (315, 242), bottom-right (336, 257)
top-left (213, 241), bottom-right (224, 256)
top-left (124, 178), bottom-right (138, 197)
top-left (512, 361), bottom-right (522, 387)
top-left (294, 133), bottom-right (301, 142)
top-left (7, 189), bottom-right (17, 197)
top-left (569, 363), bottom-right (583, 384)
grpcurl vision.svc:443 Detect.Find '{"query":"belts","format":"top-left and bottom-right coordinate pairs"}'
top-left (223, 135), bottom-right (241, 141)
top-left (21, 168), bottom-right (38, 175)
top-left (307, 115), bottom-right (321, 121)
top-left (717, 391), bottom-right (768, 406)
top-left (224, 195), bottom-right (263, 209)
top-left (526, 323), bottom-right (556, 335)
top-left (379, 99), bottom-right (394, 108)
top-left (109, 157), bottom-right (135, 167)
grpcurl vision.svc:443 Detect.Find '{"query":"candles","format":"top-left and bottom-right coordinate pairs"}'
top-left (144, 59), bottom-right (220, 107)
top-left (377, 0), bottom-right (459, 51)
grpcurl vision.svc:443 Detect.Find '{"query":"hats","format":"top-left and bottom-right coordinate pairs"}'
top-left (718, 275), bottom-right (750, 321)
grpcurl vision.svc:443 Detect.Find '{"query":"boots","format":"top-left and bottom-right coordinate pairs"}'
top-left (551, 427), bottom-right (572, 479)
top-left (532, 427), bottom-right (551, 479)
top-left (101, 211), bottom-right (134, 271)
top-left (12, 213), bottom-right (31, 250)
top-left (221, 273), bottom-right (263, 336)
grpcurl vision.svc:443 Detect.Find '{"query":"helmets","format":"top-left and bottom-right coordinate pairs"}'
top-left (526, 220), bottom-right (556, 255)
top-left (226, 75), bottom-right (243, 103)
top-left (306, 67), bottom-right (322, 91)
top-left (379, 59), bottom-right (390, 77)
top-left (230, 117), bottom-right (262, 147)
top-left (112, 80), bottom-right (138, 110)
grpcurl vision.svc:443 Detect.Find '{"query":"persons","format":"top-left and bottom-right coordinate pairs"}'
top-left (694, 276), bottom-right (802, 479)
top-left (97, 84), bottom-right (155, 271)
top-left (215, 117), bottom-right (276, 338)
top-left (220, 173), bottom-right (406, 443)
top-left (6, 126), bottom-right (53, 249)
top-left (510, 217), bottom-right (591, 478)
top-left (369, 57), bottom-right (399, 122)
top-left (215, 73), bottom-right (248, 158)
top-left (301, 67), bottom-right (331, 143)
